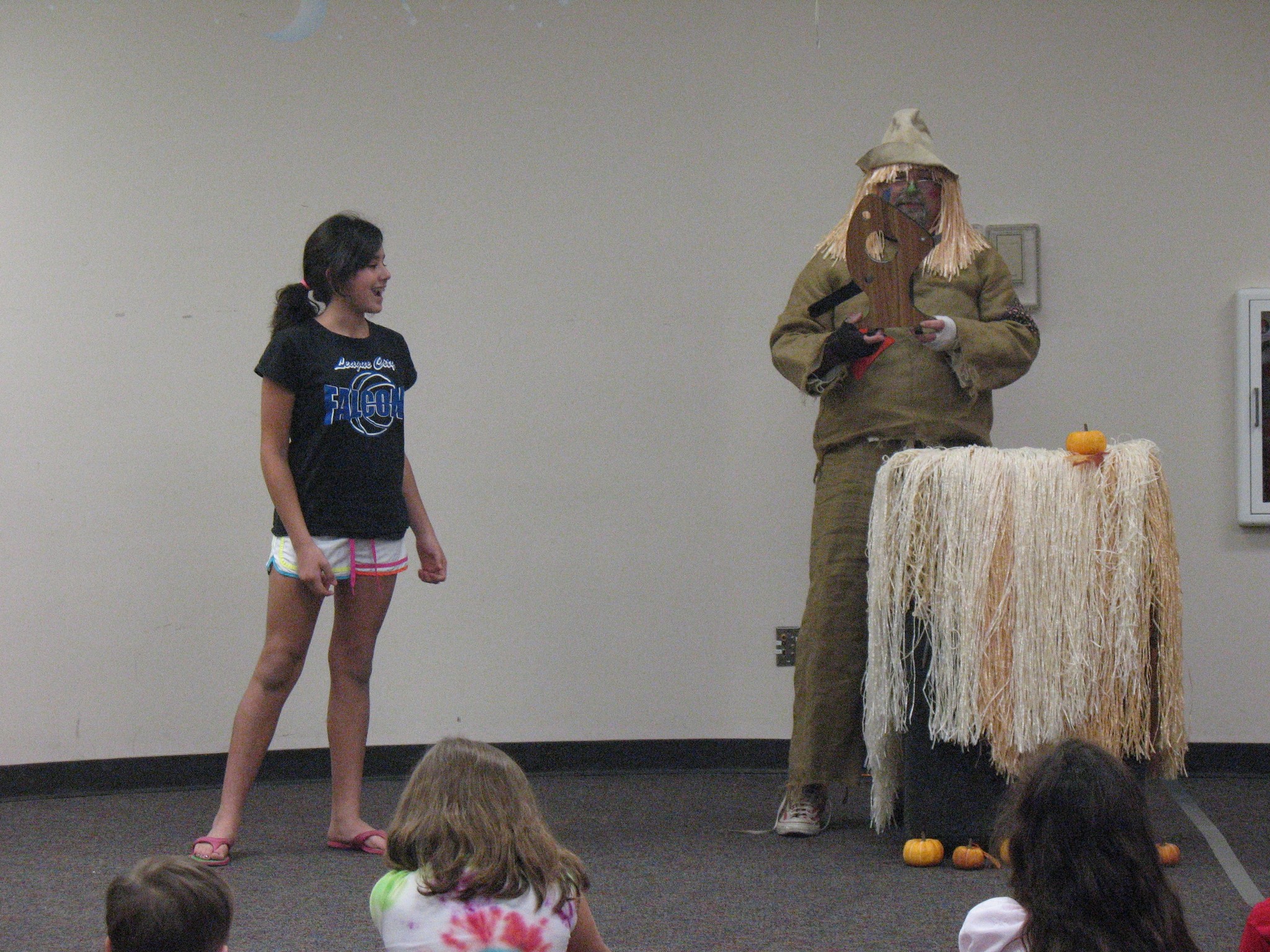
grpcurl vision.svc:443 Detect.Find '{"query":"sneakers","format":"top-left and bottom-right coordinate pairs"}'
top-left (776, 787), bottom-right (824, 838)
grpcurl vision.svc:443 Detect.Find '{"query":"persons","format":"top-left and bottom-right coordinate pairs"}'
top-left (104, 854), bottom-right (233, 952)
top-left (952, 741), bottom-right (1202, 952)
top-left (367, 738), bottom-right (613, 951)
top-left (766, 108), bottom-right (1043, 837)
top-left (187, 213), bottom-right (449, 865)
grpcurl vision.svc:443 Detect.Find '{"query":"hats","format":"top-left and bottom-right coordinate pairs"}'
top-left (854, 107), bottom-right (962, 180)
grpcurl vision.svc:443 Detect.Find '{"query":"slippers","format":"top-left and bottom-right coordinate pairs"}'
top-left (188, 834), bottom-right (236, 865)
top-left (325, 826), bottom-right (395, 856)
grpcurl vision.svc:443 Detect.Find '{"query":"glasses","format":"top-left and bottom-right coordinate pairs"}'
top-left (878, 175), bottom-right (940, 194)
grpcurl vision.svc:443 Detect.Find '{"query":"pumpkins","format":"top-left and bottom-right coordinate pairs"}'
top-left (902, 832), bottom-right (945, 866)
top-left (1000, 838), bottom-right (1014, 867)
top-left (1065, 424), bottom-right (1108, 454)
top-left (951, 838), bottom-right (984, 868)
top-left (1155, 838), bottom-right (1181, 866)
